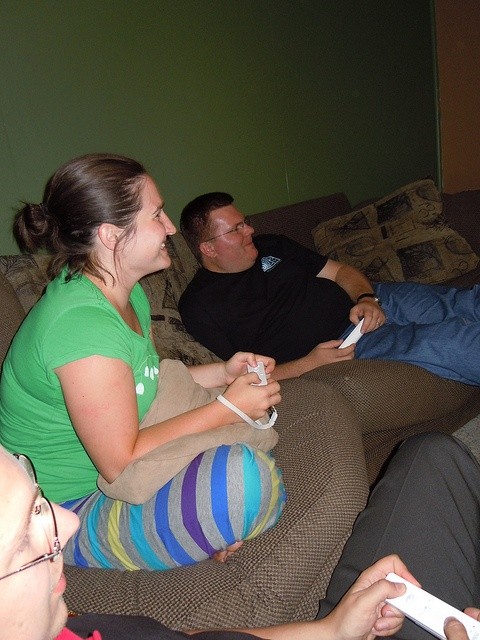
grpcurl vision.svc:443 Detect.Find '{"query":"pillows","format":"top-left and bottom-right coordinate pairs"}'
top-left (7, 233), bottom-right (223, 365)
top-left (313, 180), bottom-right (480, 284)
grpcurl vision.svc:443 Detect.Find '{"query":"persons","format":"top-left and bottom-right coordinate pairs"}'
top-left (0, 151), bottom-right (289, 577)
top-left (0, 433), bottom-right (480, 640)
top-left (177, 189), bottom-right (480, 384)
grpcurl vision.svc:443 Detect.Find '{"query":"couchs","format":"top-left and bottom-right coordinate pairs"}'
top-left (0, 192), bottom-right (480, 638)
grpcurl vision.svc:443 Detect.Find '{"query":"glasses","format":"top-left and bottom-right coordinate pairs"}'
top-left (1, 452), bottom-right (63, 579)
top-left (203, 216), bottom-right (251, 243)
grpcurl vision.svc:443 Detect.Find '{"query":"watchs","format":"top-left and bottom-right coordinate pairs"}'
top-left (354, 291), bottom-right (384, 305)
